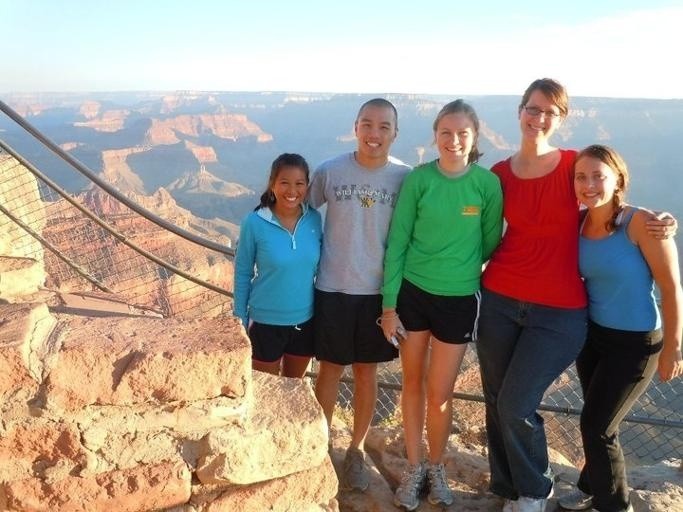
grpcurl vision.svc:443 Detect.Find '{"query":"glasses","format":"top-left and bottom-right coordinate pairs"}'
top-left (523, 105), bottom-right (561, 117)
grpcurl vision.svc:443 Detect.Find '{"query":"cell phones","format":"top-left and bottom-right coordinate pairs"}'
top-left (391, 335), bottom-right (401, 350)
top-left (396, 327), bottom-right (409, 340)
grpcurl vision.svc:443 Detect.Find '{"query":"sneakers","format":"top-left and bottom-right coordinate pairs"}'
top-left (392, 463), bottom-right (424, 511)
top-left (423, 463), bottom-right (454, 506)
top-left (499, 486), bottom-right (555, 512)
top-left (557, 486), bottom-right (595, 510)
top-left (343, 446), bottom-right (369, 493)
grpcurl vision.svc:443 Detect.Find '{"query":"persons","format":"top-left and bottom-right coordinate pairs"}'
top-left (490, 77), bottom-right (679, 511)
top-left (380, 97), bottom-right (506, 512)
top-left (232, 97), bottom-right (414, 494)
top-left (556, 143), bottom-right (683, 512)
top-left (232, 153), bottom-right (325, 379)
